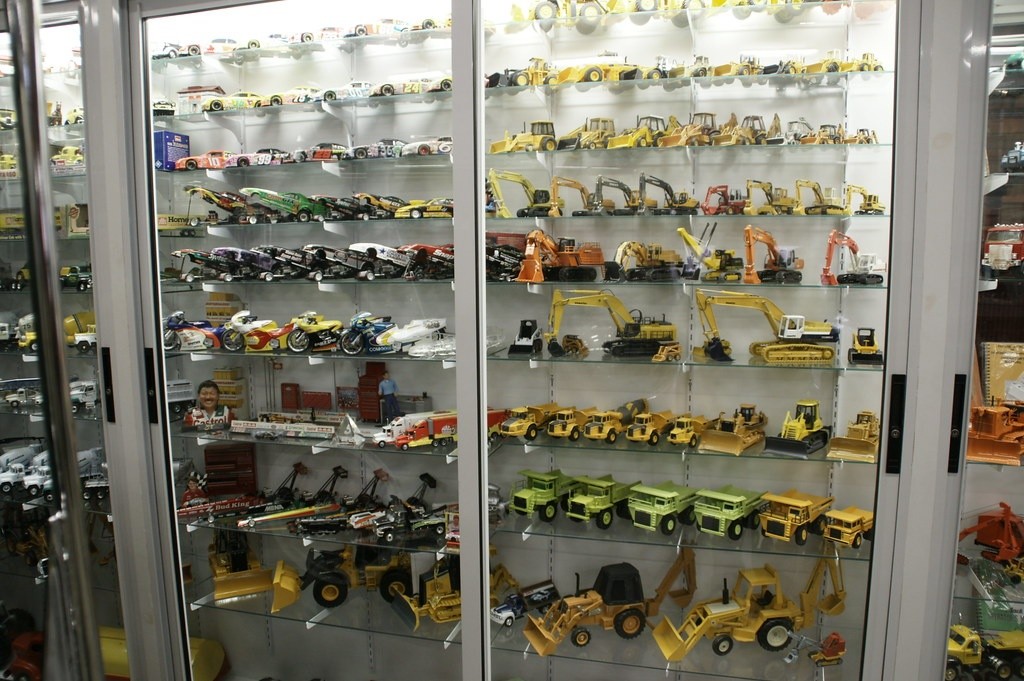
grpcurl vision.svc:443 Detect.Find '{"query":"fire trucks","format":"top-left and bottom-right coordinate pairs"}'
top-left (982, 222), bottom-right (1024, 273)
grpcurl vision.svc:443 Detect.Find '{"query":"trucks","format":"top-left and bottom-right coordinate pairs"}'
top-left (370, 410), bottom-right (460, 450)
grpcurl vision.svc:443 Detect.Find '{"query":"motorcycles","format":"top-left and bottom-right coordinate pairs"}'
top-left (162, 309), bottom-right (455, 362)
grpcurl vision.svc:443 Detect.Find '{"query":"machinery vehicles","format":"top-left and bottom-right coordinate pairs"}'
top-left (1001, 142), bottom-right (1024, 172)
top-left (241, 461), bottom-right (444, 547)
top-left (205, 552), bottom-right (470, 638)
top-left (955, 502), bottom-right (1024, 583)
top-left (944, 611), bottom-right (1024, 681)
top-left (966, 398), bottom-right (1024, 468)
top-left (1, 258), bottom-right (112, 514)
top-left (479, 1), bottom-right (901, 665)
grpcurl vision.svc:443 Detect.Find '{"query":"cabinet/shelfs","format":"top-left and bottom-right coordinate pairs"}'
top-left (0, 0), bottom-right (1024, 680)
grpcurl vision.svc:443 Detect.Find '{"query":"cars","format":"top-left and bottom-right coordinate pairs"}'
top-left (0, 56), bottom-right (87, 172)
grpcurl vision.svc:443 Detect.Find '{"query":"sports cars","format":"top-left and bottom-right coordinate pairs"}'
top-left (152, 16), bottom-right (454, 287)
